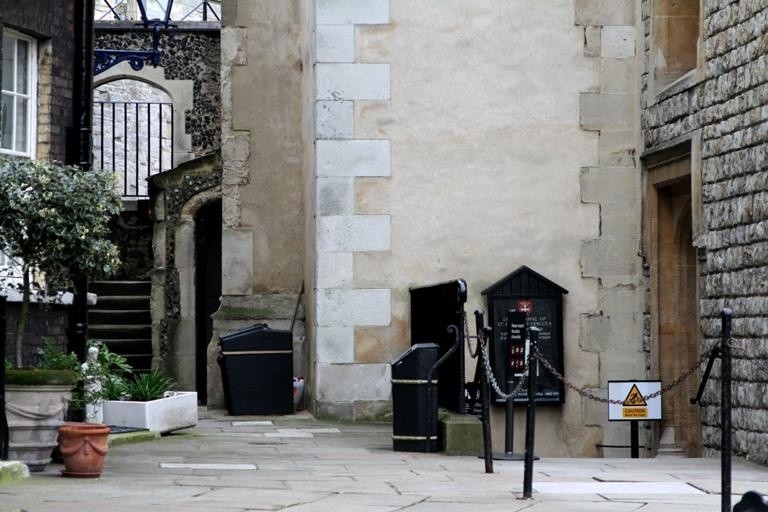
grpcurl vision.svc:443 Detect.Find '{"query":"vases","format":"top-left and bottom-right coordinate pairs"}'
top-left (56, 424), bottom-right (110, 478)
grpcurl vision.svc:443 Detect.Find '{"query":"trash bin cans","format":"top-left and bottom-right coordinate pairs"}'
top-left (217, 324), bottom-right (295, 417)
top-left (390, 343), bottom-right (440, 454)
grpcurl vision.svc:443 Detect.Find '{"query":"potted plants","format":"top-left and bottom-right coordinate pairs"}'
top-left (102, 369), bottom-right (198, 437)
top-left (4, 366), bottom-right (79, 472)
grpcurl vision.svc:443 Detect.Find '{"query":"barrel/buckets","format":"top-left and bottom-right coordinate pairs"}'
top-left (293, 377), bottom-right (304, 410)
